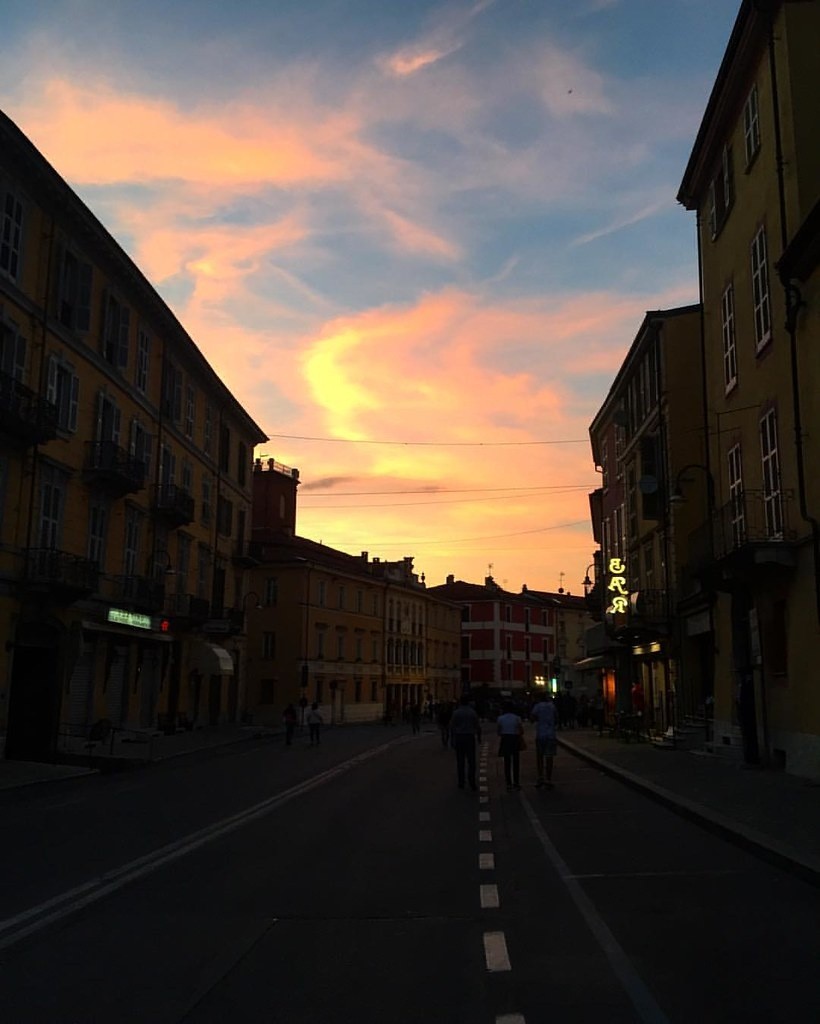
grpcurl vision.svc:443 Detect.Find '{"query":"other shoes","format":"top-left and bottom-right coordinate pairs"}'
top-left (534, 777), bottom-right (544, 789)
top-left (514, 783), bottom-right (522, 790)
top-left (506, 784), bottom-right (512, 793)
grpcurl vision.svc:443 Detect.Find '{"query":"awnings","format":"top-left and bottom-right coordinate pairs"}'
top-left (199, 647), bottom-right (234, 675)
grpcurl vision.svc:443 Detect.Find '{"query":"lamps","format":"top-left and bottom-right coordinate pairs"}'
top-left (242, 590), bottom-right (264, 610)
top-left (668, 464), bottom-right (716, 515)
top-left (147, 550), bottom-right (176, 575)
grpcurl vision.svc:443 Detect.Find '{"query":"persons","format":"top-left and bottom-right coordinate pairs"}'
top-left (402, 700), bottom-right (459, 735)
top-left (553, 689), bottom-right (605, 737)
top-left (282, 702), bottom-right (299, 746)
top-left (306, 703), bottom-right (324, 746)
top-left (530, 692), bottom-right (558, 790)
top-left (438, 703), bottom-right (452, 747)
top-left (450, 694), bottom-right (482, 792)
top-left (384, 699), bottom-right (396, 727)
top-left (495, 701), bottom-right (525, 793)
